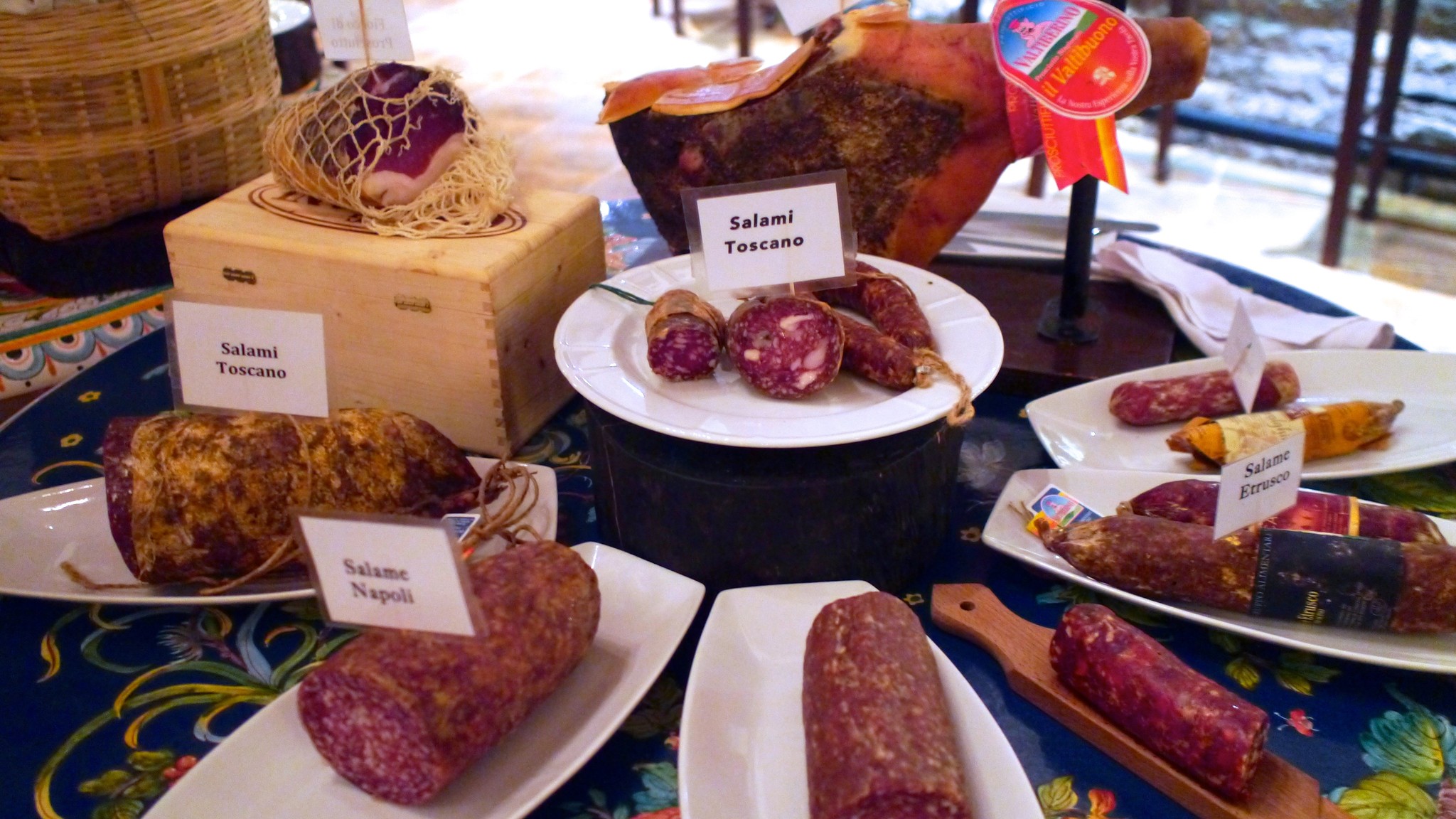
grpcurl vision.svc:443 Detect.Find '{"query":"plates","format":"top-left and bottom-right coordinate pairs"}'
top-left (929, 583), bottom-right (1354, 819)
top-left (678, 578), bottom-right (1047, 819)
top-left (553, 251), bottom-right (1006, 450)
top-left (134, 542), bottom-right (707, 819)
top-left (1024, 349), bottom-right (1456, 477)
top-left (0, 454), bottom-right (558, 602)
top-left (979, 469), bottom-right (1456, 679)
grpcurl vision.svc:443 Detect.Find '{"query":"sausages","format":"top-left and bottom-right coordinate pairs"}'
top-left (98, 0), bottom-right (1455, 819)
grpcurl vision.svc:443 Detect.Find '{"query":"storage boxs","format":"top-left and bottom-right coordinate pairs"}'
top-left (162, 170), bottom-right (608, 462)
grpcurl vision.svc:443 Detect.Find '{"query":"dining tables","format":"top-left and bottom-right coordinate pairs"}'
top-left (1, 228), bottom-right (1456, 819)
